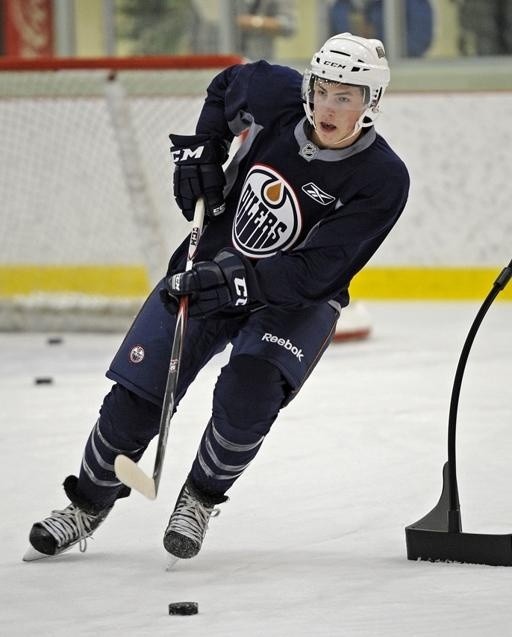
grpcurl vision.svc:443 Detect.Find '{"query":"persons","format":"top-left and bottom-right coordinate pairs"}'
top-left (222, 0), bottom-right (296, 62)
top-left (29, 30), bottom-right (410, 560)
top-left (327, 0), bottom-right (432, 59)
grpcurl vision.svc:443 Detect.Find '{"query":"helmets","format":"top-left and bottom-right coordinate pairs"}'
top-left (299, 30), bottom-right (392, 130)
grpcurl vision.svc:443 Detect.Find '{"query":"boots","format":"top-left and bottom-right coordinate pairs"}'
top-left (28, 474), bottom-right (131, 556)
top-left (162, 467), bottom-right (232, 560)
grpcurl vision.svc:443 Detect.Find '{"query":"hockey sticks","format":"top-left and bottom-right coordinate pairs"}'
top-left (114, 198), bottom-right (207, 500)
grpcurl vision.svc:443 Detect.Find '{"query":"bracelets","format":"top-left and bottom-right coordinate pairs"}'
top-left (252, 14), bottom-right (263, 29)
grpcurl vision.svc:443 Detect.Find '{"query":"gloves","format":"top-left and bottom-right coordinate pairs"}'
top-left (168, 129), bottom-right (232, 224)
top-left (158, 247), bottom-right (265, 324)
